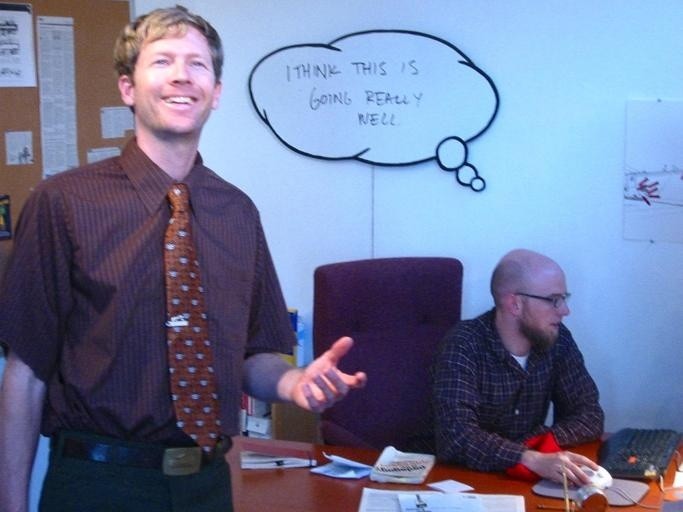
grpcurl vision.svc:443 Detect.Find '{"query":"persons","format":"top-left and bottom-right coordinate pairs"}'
top-left (0, 3), bottom-right (368, 511)
top-left (422, 247), bottom-right (605, 491)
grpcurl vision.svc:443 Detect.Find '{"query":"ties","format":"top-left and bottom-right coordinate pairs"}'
top-left (164, 186), bottom-right (221, 455)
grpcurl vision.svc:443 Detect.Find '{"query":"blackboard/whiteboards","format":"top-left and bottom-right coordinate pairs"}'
top-left (132, 2), bottom-right (683, 433)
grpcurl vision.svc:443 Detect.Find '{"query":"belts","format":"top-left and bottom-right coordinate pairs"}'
top-left (61, 437), bottom-right (229, 474)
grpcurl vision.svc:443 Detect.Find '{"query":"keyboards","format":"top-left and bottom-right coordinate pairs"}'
top-left (598, 427), bottom-right (680, 480)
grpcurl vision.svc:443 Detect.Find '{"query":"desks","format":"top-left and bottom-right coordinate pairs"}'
top-left (223, 431), bottom-right (683, 512)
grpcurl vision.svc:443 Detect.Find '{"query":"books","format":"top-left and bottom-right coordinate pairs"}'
top-left (368, 446), bottom-right (435, 484)
top-left (238, 448), bottom-right (318, 468)
top-left (308, 449), bottom-right (373, 479)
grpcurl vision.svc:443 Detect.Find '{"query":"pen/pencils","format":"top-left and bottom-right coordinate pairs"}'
top-left (537, 467), bottom-right (570, 512)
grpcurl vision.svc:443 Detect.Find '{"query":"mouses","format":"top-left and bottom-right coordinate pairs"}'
top-left (571, 463), bottom-right (614, 490)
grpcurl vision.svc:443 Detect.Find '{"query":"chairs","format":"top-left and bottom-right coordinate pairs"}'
top-left (313, 257), bottom-right (463, 456)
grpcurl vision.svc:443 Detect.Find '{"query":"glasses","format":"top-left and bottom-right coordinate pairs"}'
top-left (517, 293), bottom-right (570, 307)
